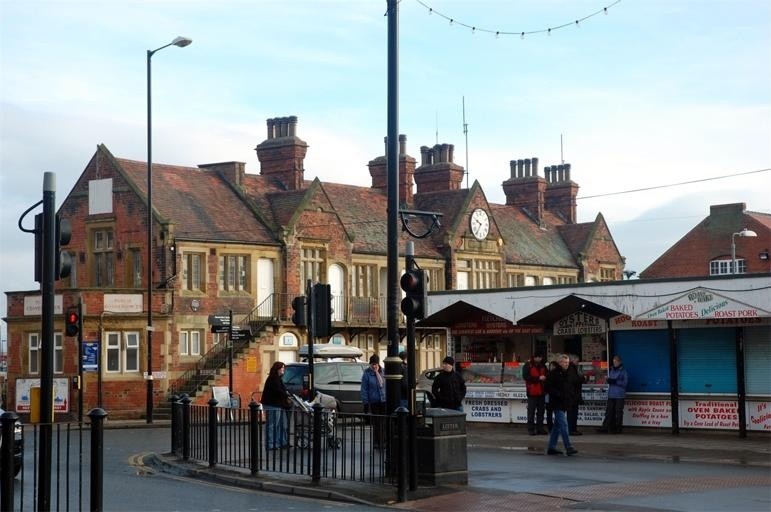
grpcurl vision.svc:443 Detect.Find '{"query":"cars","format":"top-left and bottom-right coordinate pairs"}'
top-left (0, 406), bottom-right (24, 480)
top-left (417, 367), bottom-right (445, 393)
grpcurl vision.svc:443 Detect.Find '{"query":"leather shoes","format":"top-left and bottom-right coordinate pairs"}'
top-left (570, 431), bottom-right (583, 435)
top-left (567, 447), bottom-right (578, 454)
top-left (529, 429), bottom-right (536, 435)
top-left (548, 448), bottom-right (564, 455)
top-left (538, 428), bottom-right (548, 434)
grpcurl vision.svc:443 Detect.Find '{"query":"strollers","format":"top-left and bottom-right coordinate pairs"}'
top-left (286, 389), bottom-right (344, 450)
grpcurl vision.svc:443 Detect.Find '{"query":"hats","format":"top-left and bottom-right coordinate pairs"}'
top-left (370, 354), bottom-right (379, 364)
top-left (443, 356), bottom-right (455, 366)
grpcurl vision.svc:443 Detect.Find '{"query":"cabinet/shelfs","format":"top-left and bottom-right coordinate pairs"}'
top-left (502, 362), bottom-right (608, 426)
top-left (454, 362), bottom-right (510, 423)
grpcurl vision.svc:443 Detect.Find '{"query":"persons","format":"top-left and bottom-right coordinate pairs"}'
top-left (261, 362), bottom-right (293, 450)
top-left (522, 351), bottom-right (548, 435)
top-left (400, 350), bottom-right (411, 409)
top-left (433, 356), bottom-right (467, 411)
top-left (360, 354), bottom-right (385, 449)
top-left (543, 354), bottom-right (579, 455)
top-left (597, 354), bottom-right (629, 433)
top-left (568, 354), bottom-right (586, 435)
top-left (547, 361), bottom-right (560, 433)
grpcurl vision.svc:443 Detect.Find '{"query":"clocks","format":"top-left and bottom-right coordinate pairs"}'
top-left (470, 207), bottom-right (489, 242)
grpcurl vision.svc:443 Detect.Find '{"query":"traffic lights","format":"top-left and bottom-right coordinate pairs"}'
top-left (65, 308), bottom-right (79, 338)
top-left (292, 293), bottom-right (308, 331)
top-left (400, 270), bottom-right (428, 321)
top-left (32, 214), bottom-right (73, 284)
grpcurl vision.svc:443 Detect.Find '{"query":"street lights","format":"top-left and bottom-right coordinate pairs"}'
top-left (731, 226), bottom-right (760, 274)
top-left (142, 34), bottom-right (195, 427)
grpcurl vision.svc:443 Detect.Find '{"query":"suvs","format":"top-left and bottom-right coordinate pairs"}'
top-left (275, 342), bottom-right (386, 427)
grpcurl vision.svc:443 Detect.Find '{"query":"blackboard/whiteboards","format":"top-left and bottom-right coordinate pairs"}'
top-left (211, 386), bottom-right (230, 408)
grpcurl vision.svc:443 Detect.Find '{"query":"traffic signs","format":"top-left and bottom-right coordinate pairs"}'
top-left (205, 313), bottom-right (251, 341)
